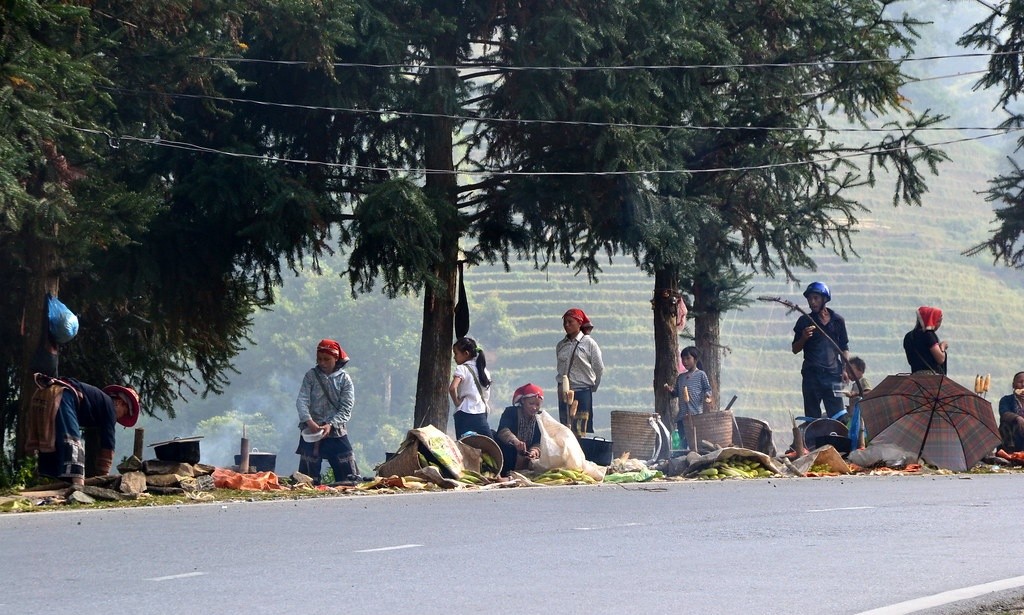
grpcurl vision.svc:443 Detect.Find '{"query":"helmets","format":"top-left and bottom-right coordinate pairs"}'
top-left (803, 282), bottom-right (832, 302)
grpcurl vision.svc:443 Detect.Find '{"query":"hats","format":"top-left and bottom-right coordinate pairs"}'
top-left (102, 384), bottom-right (141, 427)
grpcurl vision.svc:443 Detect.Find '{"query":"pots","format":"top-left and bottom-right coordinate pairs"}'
top-left (154, 436), bottom-right (200, 467)
top-left (234, 448), bottom-right (277, 474)
top-left (578, 435), bottom-right (614, 466)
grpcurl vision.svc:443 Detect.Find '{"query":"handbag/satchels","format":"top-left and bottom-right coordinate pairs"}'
top-left (454, 261), bottom-right (470, 340)
top-left (46, 290), bottom-right (80, 344)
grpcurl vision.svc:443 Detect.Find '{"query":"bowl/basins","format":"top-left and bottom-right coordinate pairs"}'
top-left (301, 427), bottom-right (325, 443)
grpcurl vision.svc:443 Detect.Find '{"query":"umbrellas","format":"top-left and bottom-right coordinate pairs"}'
top-left (857, 372), bottom-right (1004, 472)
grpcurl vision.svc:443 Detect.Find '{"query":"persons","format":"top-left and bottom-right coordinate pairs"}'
top-left (555, 308), bottom-right (605, 434)
top-left (295, 339), bottom-right (360, 481)
top-left (491, 384), bottom-right (541, 477)
top-left (845, 357), bottom-right (873, 448)
top-left (998, 372), bottom-right (1024, 453)
top-left (448, 338), bottom-right (494, 440)
top-left (902, 306), bottom-right (949, 375)
top-left (792, 282), bottom-right (848, 422)
top-left (664, 346), bottom-right (712, 449)
top-left (25, 376), bottom-right (140, 486)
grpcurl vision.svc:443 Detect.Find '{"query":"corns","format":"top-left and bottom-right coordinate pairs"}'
top-left (531, 467), bottom-right (597, 485)
top-left (697, 454), bottom-right (772, 479)
top-left (975, 373), bottom-right (991, 393)
top-left (417, 450), bottom-right (499, 485)
top-left (683, 385), bottom-right (689, 403)
top-left (562, 374), bottom-right (579, 417)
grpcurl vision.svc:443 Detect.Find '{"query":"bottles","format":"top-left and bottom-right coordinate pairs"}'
top-left (672, 429), bottom-right (680, 450)
